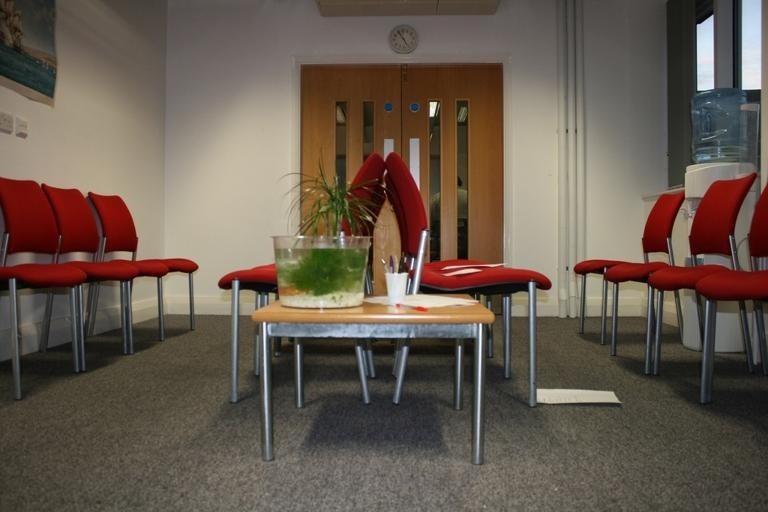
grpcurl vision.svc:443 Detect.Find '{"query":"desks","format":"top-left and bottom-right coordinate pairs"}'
top-left (251, 293), bottom-right (496, 465)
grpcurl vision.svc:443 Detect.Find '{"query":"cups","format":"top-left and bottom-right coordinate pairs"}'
top-left (384, 274), bottom-right (408, 298)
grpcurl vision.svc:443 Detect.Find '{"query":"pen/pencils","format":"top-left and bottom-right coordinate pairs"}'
top-left (381, 254), bottom-right (414, 274)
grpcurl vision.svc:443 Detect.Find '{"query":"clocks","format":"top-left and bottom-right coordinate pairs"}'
top-left (389, 24), bottom-right (418, 54)
